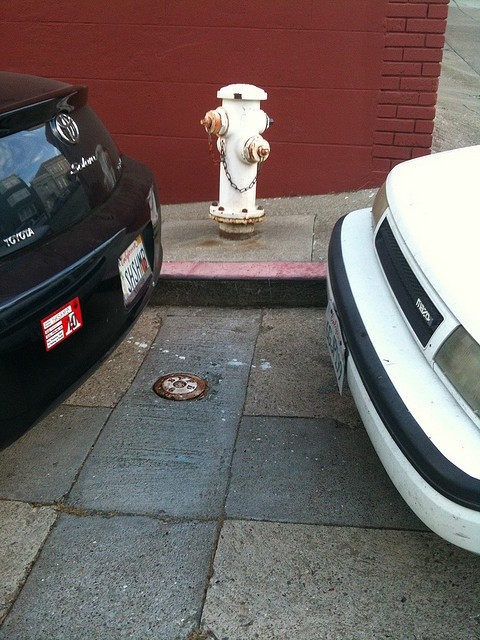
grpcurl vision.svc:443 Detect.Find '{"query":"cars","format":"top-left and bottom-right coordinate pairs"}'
top-left (325, 147), bottom-right (480, 552)
top-left (0, 71), bottom-right (164, 450)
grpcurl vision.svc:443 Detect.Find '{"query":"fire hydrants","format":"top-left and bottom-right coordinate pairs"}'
top-left (200, 84), bottom-right (274, 240)
